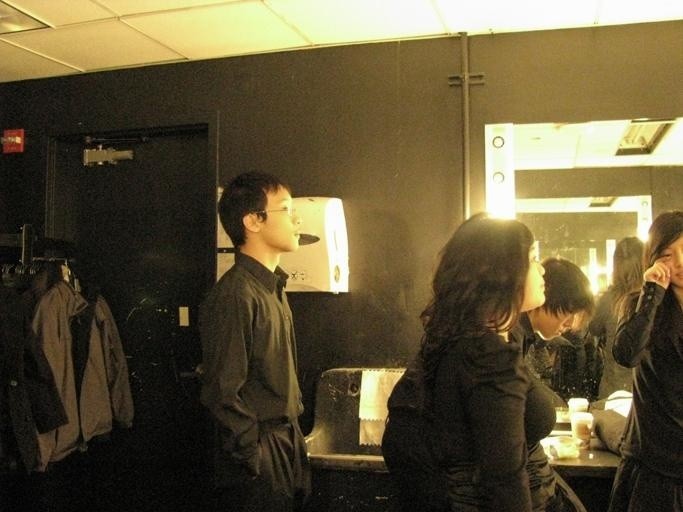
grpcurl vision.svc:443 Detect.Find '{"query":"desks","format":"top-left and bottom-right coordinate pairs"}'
top-left (539, 438), bottom-right (620, 511)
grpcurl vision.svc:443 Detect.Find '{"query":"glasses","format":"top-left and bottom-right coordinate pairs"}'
top-left (251, 206), bottom-right (297, 218)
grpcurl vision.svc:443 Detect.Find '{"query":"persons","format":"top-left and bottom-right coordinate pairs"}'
top-left (195, 170), bottom-right (313, 512)
top-left (547, 294), bottom-right (606, 401)
top-left (604, 209), bottom-right (683, 512)
top-left (587, 235), bottom-right (644, 401)
top-left (415, 210), bottom-right (561, 511)
top-left (382, 258), bottom-right (596, 510)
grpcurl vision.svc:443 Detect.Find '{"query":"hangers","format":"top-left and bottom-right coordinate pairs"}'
top-left (0, 253), bottom-right (69, 280)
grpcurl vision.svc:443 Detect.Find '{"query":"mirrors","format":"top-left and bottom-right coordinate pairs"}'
top-left (482, 120), bottom-right (682, 438)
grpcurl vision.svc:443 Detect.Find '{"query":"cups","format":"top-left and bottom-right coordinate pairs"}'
top-left (569, 411), bottom-right (594, 451)
top-left (567, 397), bottom-right (590, 414)
